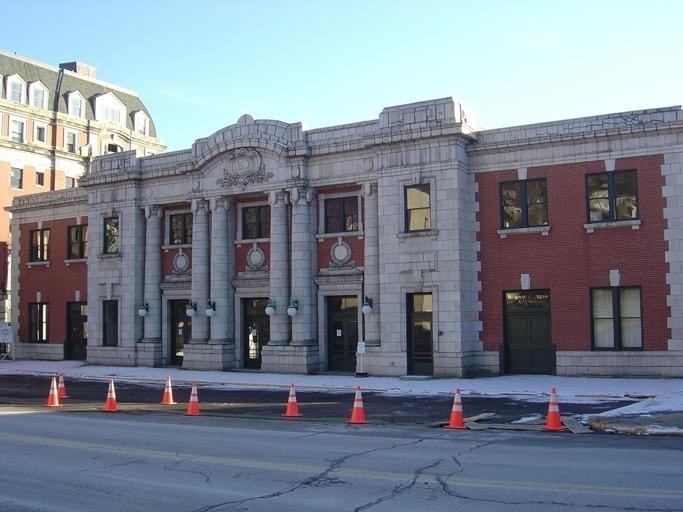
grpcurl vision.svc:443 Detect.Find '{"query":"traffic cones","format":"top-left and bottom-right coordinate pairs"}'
top-left (159, 374), bottom-right (177, 405)
top-left (540, 387), bottom-right (567, 431)
top-left (42, 376), bottom-right (63, 408)
top-left (57, 370), bottom-right (69, 399)
top-left (181, 381), bottom-right (202, 416)
top-left (280, 383), bottom-right (303, 417)
top-left (101, 378), bottom-right (122, 412)
top-left (442, 387), bottom-right (469, 429)
top-left (344, 385), bottom-right (370, 424)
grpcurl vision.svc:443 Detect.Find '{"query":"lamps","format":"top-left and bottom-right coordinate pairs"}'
top-left (205, 300), bottom-right (215, 317)
top-left (263, 299), bottom-right (277, 316)
top-left (137, 303), bottom-right (148, 317)
top-left (360, 296), bottom-right (373, 315)
top-left (286, 298), bottom-right (297, 316)
top-left (185, 301), bottom-right (196, 317)
